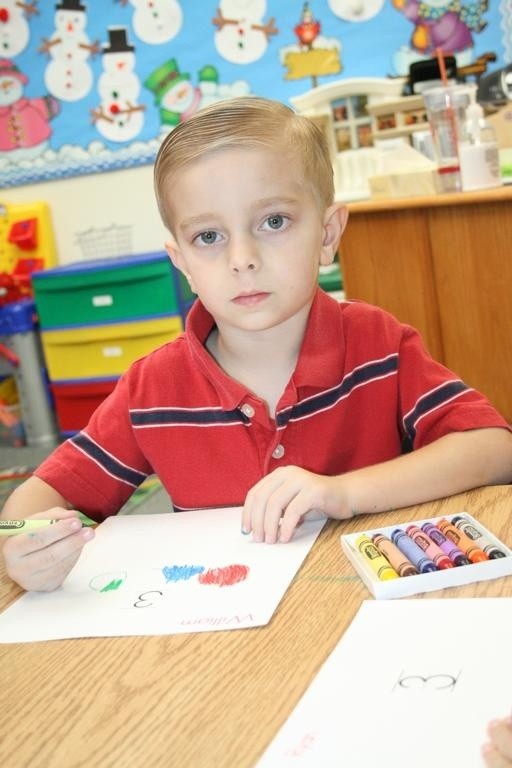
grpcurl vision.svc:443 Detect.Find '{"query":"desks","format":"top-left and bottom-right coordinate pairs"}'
top-left (1, 485), bottom-right (512, 768)
top-left (339, 183), bottom-right (512, 432)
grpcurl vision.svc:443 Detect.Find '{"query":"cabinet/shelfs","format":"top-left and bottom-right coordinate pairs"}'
top-left (31, 249), bottom-right (205, 444)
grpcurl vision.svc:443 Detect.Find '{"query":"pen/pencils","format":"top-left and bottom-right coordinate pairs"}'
top-left (0, 517), bottom-right (97, 535)
top-left (357, 516), bottom-right (506, 581)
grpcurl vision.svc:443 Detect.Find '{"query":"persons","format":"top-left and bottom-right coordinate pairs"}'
top-left (1, 95), bottom-right (512, 596)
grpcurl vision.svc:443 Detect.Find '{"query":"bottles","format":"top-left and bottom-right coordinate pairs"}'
top-left (423, 83), bottom-right (503, 193)
